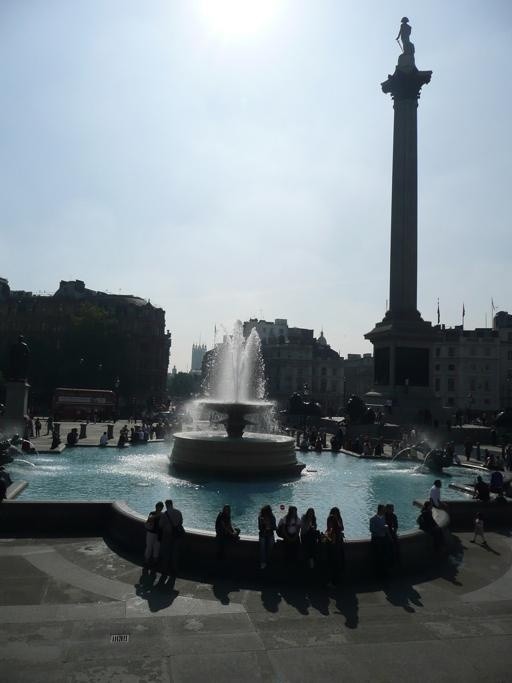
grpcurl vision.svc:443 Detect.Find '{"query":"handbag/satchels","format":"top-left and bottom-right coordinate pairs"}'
top-left (276, 525), bottom-right (287, 539)
top-left (171, 525), bottom-right (186, 538)
top-left (144, 520), bottom-right (155, 531)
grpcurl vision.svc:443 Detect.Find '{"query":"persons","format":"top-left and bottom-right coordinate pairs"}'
top-left (214, 504), bottom-right (239, 575)
top-left (369, 503), bottom-right (391, 571)
top-left (489, 468), bottom-right (505, 496)
top-left (282, 506), bottom-right (301, 573)
top-left (418, 500), bottom-right (448, 550)
top-left (469, 511), bottom-right (487, 543)
top-left (257, 503), bottom-right (277, 576)
top-left (326, 506), bottom-right (344, 569)
top-left (472, 474), bottom-right (491, 501)
top-left (289, 399), bottom-right (512, 470)
top-left (142, 501), bottom-right (163, 563)
top-left (299, 507), bottom-right (318, 568)
top-left (158, 499), bottom-right (184, 568)
top-left (0, 406), bottom-right (178, 453)
top-left (384, 503), bottom-right (401, 561)
top-left (427, 477), bottom-right (451, 513)
top-left (0, 465), bottom-right (12, 500)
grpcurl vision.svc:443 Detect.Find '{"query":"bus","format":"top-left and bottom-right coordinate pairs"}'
top-left (51, 387), bottom-right (122, 423)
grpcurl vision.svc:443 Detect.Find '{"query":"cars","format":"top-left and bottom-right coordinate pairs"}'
top-left (150, 393), bottom-right (187, 421)
